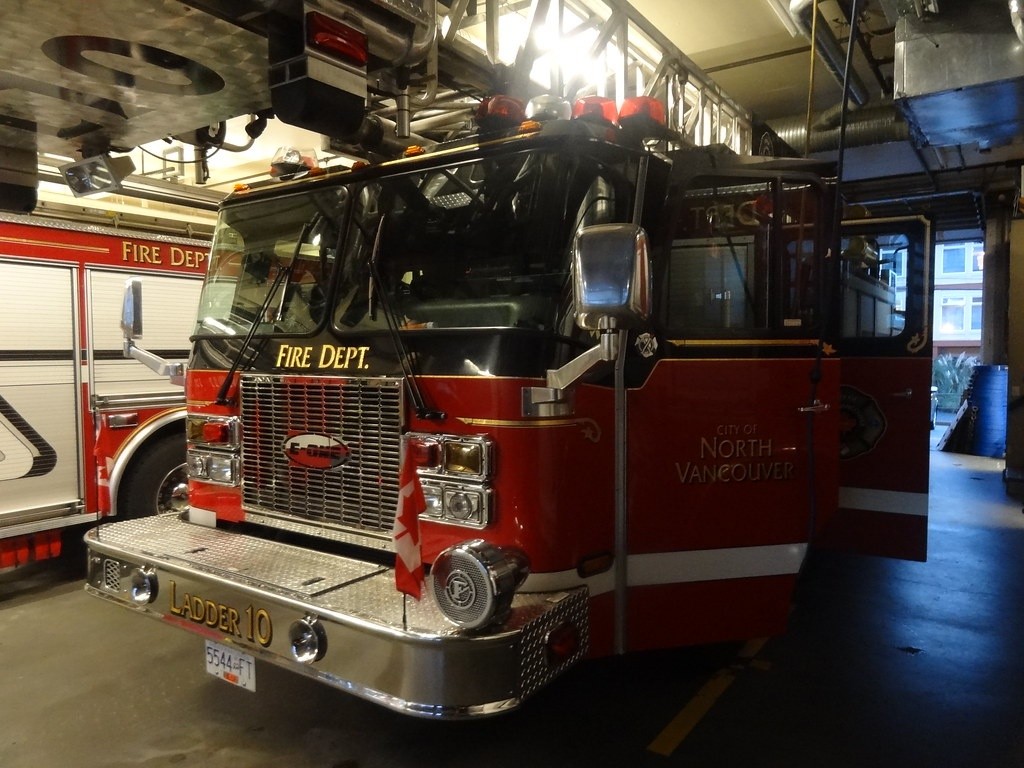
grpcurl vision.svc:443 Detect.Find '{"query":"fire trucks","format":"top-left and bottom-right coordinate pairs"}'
top-left (0, 214), bottom-right (338, 573)
top-left (81, 88), bottom-right (937, 725)
